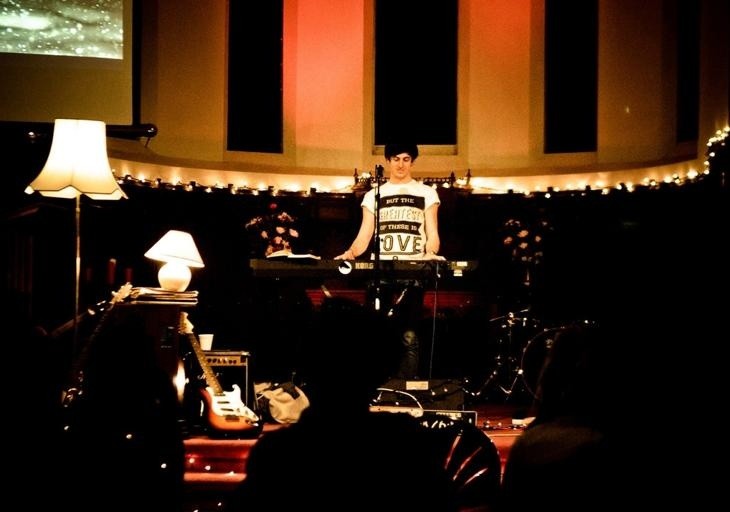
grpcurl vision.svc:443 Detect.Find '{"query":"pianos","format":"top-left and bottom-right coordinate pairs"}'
top-left (250, 258), bottom-right (480, 278)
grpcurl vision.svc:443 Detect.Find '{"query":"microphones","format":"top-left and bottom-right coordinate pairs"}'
top-left (375, 164), bottom-right (385, 179)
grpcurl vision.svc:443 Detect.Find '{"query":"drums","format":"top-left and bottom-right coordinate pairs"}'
top-left (521, 327), bottom-right (607, 405)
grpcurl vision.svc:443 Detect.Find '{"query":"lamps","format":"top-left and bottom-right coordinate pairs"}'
top-left (24, 119), bottom-right (128, 405)
top-left (144, 229), bottom-right (205, 293)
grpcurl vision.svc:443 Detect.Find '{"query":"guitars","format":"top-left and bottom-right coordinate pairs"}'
top-left (61, 282), bottom-right (134, 410)
top-left (179, 312), bottom-right (261, 438)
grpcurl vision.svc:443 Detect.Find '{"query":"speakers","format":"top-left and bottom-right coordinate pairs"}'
top-left (192, 351), bottom-right (249, 426)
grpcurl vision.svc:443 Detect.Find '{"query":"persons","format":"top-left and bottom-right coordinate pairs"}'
top-left (333, 139), bottom-right (442, 311)
top-left (1, 272), bottom-right (730, 511)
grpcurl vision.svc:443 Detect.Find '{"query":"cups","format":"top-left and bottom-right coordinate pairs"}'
top-left (198, 333), bottom-right (214, 352)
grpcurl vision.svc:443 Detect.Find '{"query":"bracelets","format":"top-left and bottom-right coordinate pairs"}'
top-left (429, 251), bottom-right (437, 255)
